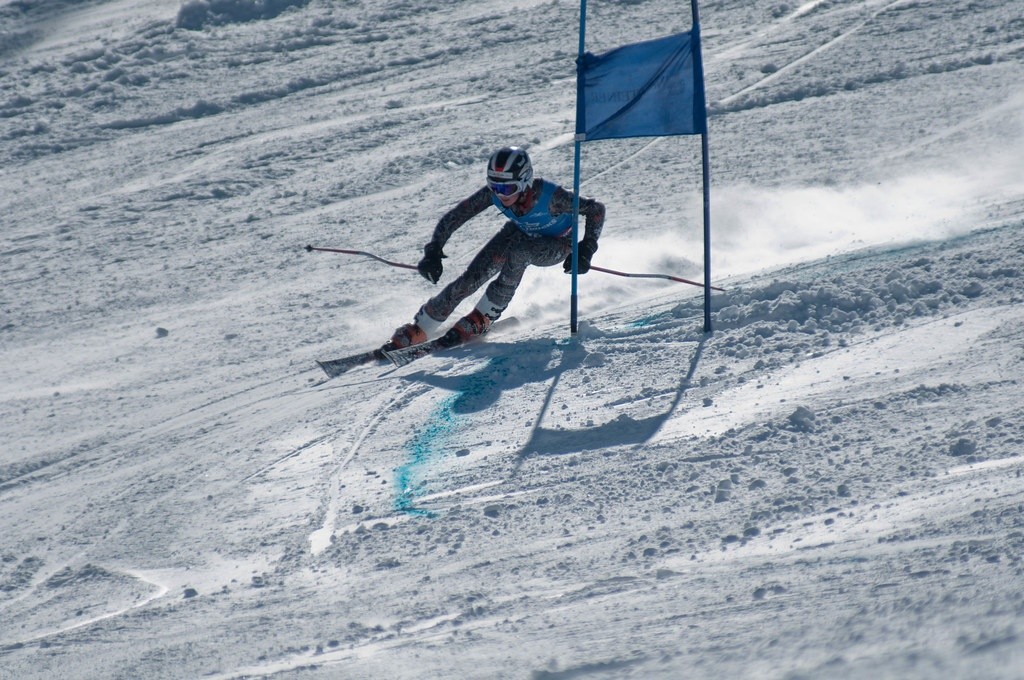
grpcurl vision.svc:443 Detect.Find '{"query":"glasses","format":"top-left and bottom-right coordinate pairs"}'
top-left (490, 183), bottom-right (521, 197)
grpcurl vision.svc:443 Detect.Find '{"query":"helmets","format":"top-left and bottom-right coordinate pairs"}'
top-left (486, 146), bottom-right (533, 191)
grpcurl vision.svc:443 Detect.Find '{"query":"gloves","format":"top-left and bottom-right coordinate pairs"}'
top-left (418, 242), bottom-right (443, 284)
top-left (563, 238), bottom-right (598, 274)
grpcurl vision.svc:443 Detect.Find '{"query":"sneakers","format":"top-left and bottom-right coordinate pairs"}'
top-left (386, 304), bottom-right (444, 351)
top-left (444, 290), bottom-right (508, 346)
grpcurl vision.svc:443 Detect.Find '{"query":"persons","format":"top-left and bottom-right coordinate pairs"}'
top-left (391, 146), bottom-right (606, 350)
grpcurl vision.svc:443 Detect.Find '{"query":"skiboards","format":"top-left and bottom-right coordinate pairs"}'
top-left (317, 316), bottom-right (521, 379)
